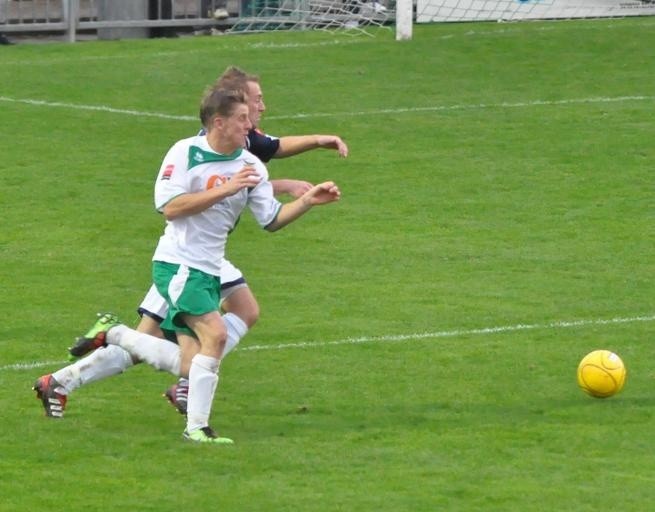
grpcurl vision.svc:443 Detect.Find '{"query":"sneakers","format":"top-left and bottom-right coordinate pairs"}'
top-left (182, 425), bottom-right (234, 445)
top-left (68, 312), bottom-right (122, 357)
top-left (165, 377), bottom-right (188, 414)
top-left (32, 374), bottom-right (68, 417)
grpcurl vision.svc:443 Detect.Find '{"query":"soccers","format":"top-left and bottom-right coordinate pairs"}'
top-left (577, 350), bottom-right (626, 398)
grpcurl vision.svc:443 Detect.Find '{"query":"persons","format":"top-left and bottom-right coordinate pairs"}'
top-left (67, 85), bottom-right (342, 444)
top-left (32, 66), bottom-right (348, 427)
